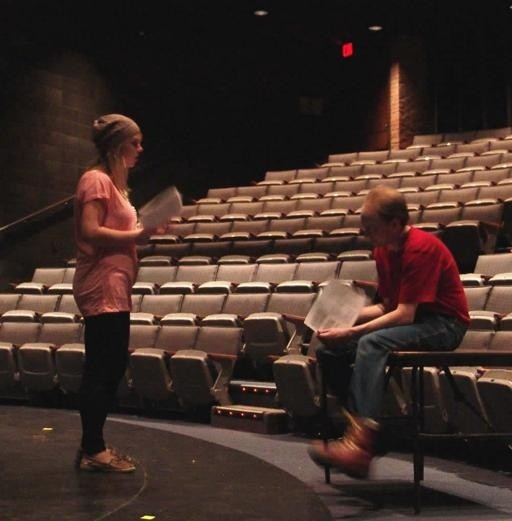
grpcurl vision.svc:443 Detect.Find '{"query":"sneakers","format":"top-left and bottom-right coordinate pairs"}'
top-left (307, 417), bottom-right (375, 480)
top-left (76, 446), bottom-right (131, 462)
top-left (82, 456), bottom-right (135, 471)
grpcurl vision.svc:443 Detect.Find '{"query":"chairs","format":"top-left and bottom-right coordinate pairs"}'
top-left (0, 127), bottom-right (512, 434)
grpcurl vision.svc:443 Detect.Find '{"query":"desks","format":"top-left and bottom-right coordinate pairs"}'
top-left (313, 348), bottom-right (512, 515)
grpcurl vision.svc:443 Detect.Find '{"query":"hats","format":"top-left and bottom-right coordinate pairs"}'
top-left (91, 115), bottom-right (141, 153)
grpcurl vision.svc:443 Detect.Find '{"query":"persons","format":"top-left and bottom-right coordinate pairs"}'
top-left (308, 188), bottom-right (470, 476)
top-left (72, 113), bottom-right (156, 473)
